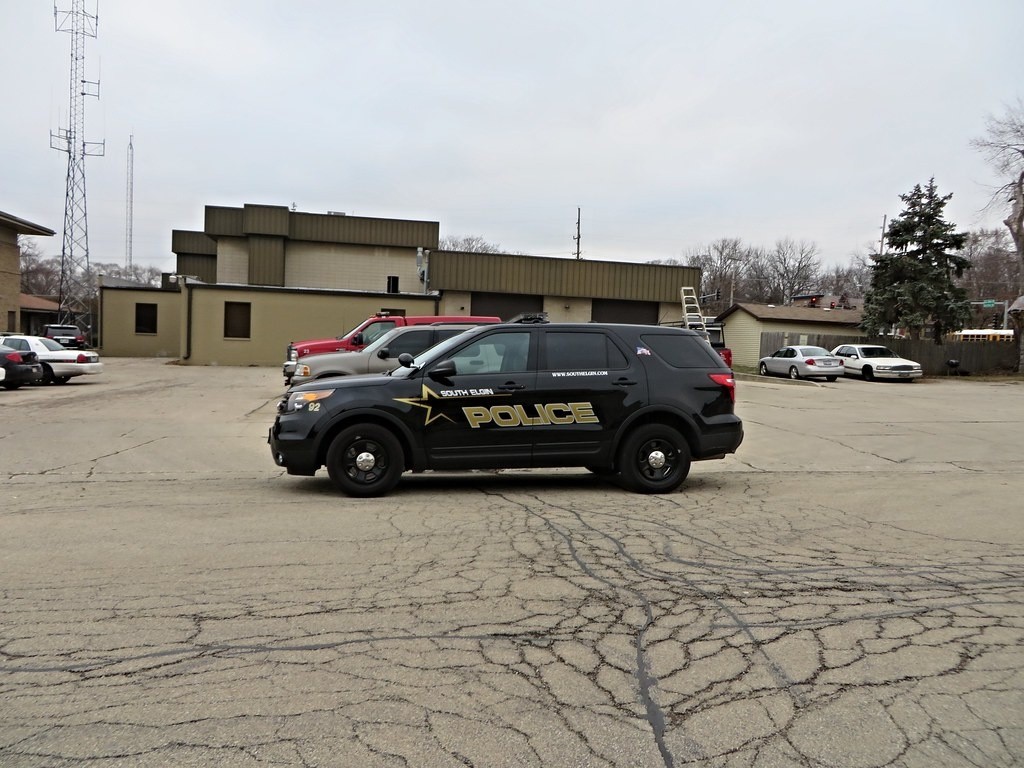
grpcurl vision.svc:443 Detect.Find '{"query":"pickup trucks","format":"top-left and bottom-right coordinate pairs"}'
top-left (282, 308), bottom-right (504, 386)
top-left (680, 321), bottom-right (734, 369)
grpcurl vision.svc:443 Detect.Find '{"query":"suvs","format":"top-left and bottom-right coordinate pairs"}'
top-left (33, 324), bottom-right (87, 350)
top-left (268, 310), bottom-right (745, 498)
top-left (285, 321), bottom-right (507, 390)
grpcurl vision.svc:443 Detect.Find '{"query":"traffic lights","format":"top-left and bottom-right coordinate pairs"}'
top-left (700, 293), bottom-right (706, 303)
top-left (810, 298), bottom-right (816, 308)
top-left (830, 301), bottom-right (835, 309)
top-left (715, 288), bottom-right (721, 301)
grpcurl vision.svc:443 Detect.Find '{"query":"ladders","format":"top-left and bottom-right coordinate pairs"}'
top-left (680, 286), bottom-right (712, 347)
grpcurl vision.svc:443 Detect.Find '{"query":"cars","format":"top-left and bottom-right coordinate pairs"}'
top-left (757, 345), bottom-right (845, 382)
top-left (830, 344), bottom-right (923, 384)
top-left (0, 331), bottom-right (104, 387)
top-left (0, 344), bottom-right (44, 390)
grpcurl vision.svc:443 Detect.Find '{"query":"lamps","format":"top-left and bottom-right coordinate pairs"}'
top-left (829, 301), bottom-right (835, 308)
top-left (809, 296), bottom-right (817, 307)
top-left (460, 306), bottom-right (464, 310)
top-left (168, 272), bottom-right (181, 283)
